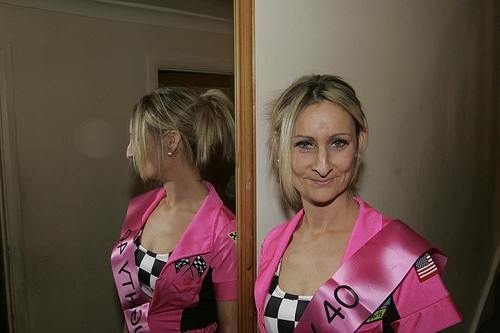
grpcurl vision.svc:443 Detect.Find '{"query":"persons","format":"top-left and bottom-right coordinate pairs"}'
top-left (107, 85), bottom-right (236, 333)
top-left (253, 72), bottom-right (464, 333)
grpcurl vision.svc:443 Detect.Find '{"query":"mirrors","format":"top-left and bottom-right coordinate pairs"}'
top-left (0, 0), bottom-right (257, 333)
